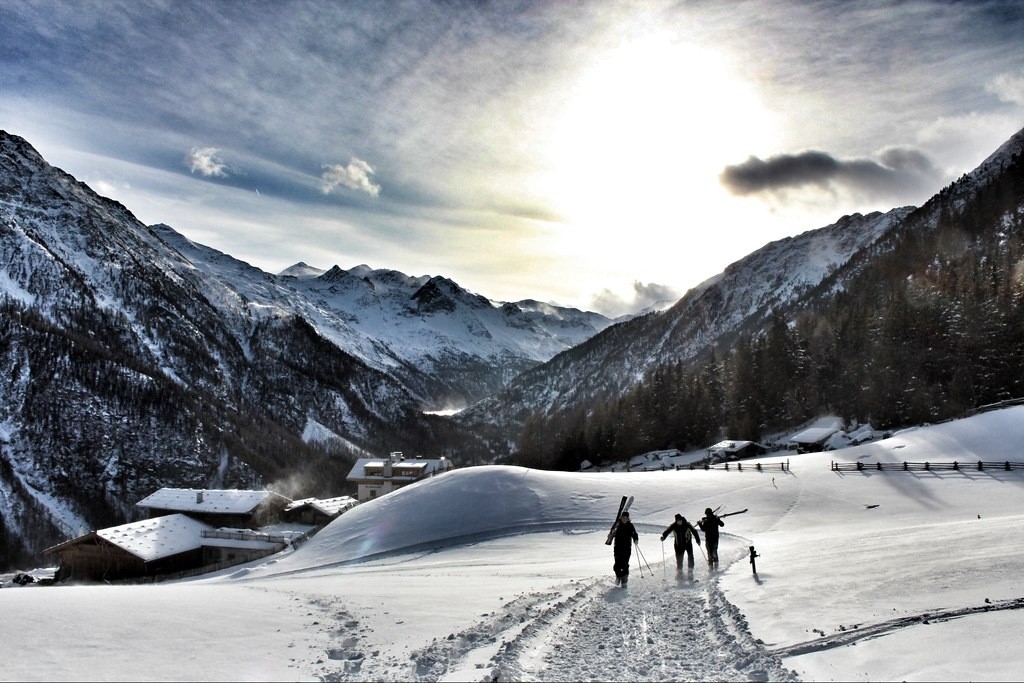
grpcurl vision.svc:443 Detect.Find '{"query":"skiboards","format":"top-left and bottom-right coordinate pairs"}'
top-left (702, 508), bottom-right (748, 521)
top-left (605, 495), bottom-right (635, 546)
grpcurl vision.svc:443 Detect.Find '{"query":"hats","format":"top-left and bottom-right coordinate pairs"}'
top-left (622, 511), bottom-right (629, 517)
top-left (705, 508), bottom-right (715, 514)
top-left (675, 514), bottom-right (683, 521)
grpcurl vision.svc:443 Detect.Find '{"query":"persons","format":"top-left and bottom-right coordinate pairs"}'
top-left (660, 514), bottom-right (701, 580)
top-left (697, 508), bottom-right (725, 573)
top-left (608, 512), bottom-right (639, 588)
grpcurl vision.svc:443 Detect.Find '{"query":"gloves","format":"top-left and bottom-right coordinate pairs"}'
top-left (697, 519), bottom-right (702, 526)
